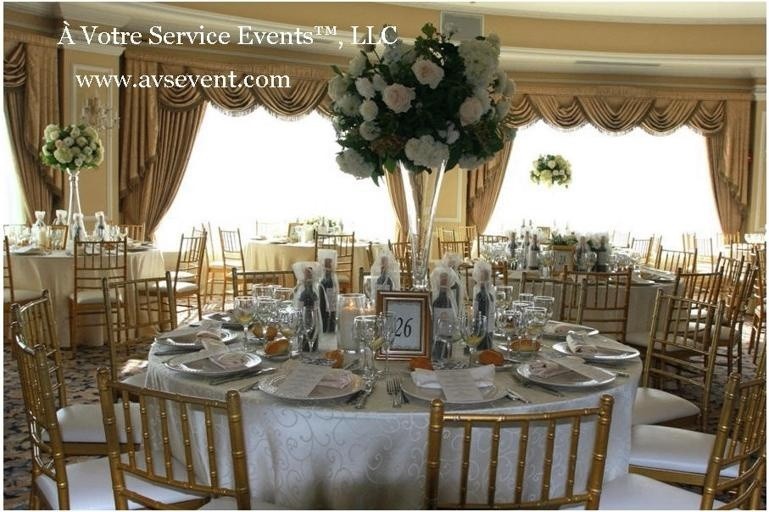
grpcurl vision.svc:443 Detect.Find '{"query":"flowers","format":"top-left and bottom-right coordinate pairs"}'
top-left (528, 153), bottom-right (575, 190)
top-left (39, 122), bottom-right (106, 175)
top-left (322, 26), bottom-right (519, 190)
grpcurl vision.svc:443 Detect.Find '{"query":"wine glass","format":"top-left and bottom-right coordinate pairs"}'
top-left (294, 224), bottom-right (342, 247)
top-left (481, 243), bottom-right (642, 276)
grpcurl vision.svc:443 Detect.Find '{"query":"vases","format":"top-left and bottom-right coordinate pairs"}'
top-left (396, 154), bottom-right (447, 290)
top-left (66, 171), bottom-right (87, 242)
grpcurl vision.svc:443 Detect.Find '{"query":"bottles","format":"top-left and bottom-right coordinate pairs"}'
top-left (597, 236), bottom-right (608, 272)
top-left (431, 275), bottom-right (452, 360)
top-left (573, 236), bottom-right (588, 272)
top-left (319, 256), bottom-right (339, 331)
top-left (448, 256), bottom-right (460, 307)
top-left (506, 218), bottom-right (541, 269)
top-left (472, 264), bottom-right (496, 350)
top-left (373, 255), bottom-right (394, 312)
top-left (319, 217), bottom-right (328, 239)
top-left (34, 209), bottom-right (106, 244)
top-left (298, 266), bottom-right (319, 351)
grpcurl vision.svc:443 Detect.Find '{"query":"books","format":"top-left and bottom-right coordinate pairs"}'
top-left (435, 369), bottom-right (485, 405)
top-left (553, 351), bottom-right (613, 385)
top-left (275, 364), bottom-right (330, 399)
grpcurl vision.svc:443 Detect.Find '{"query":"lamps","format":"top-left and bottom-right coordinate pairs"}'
top-left (79, 91), bottom-right (123, 133)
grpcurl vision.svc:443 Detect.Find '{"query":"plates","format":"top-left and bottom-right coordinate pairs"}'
top-left (153, 286), bottom-right (642, 408)
top-left (251, 236), bottom-right (380, 246)
top-left (3, 225), bottom-right (154, 256)
top-left (496, 267), bottom-right (677, 285)
